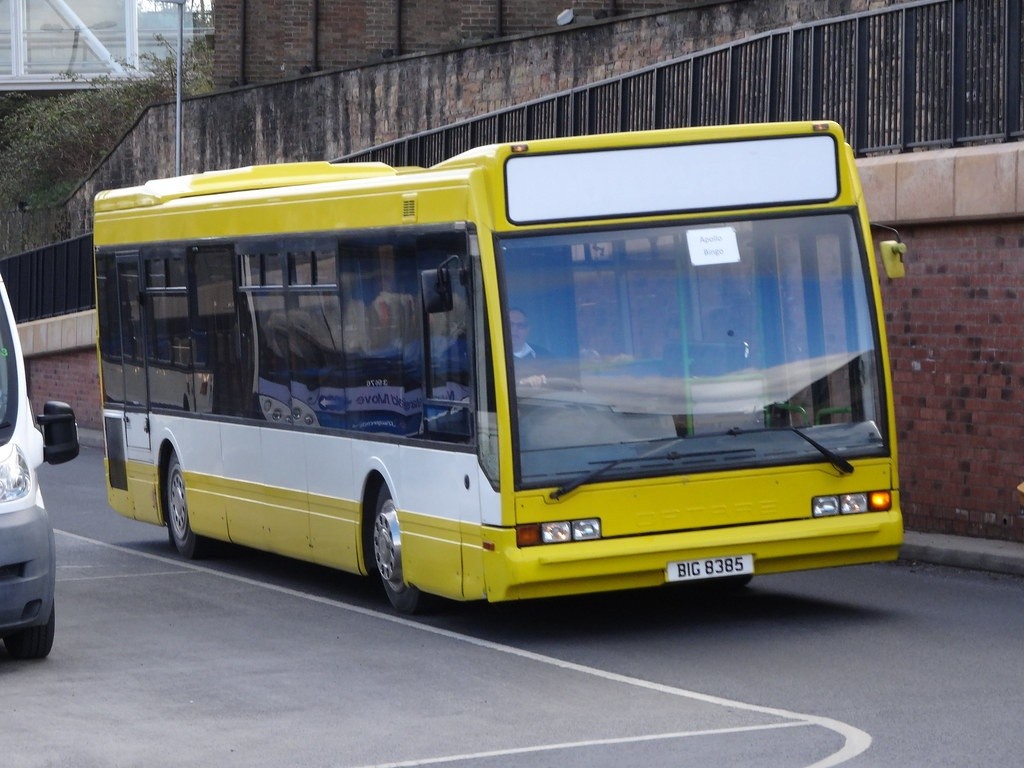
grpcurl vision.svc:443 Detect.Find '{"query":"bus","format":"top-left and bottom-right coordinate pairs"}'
top-left (93, 120), bottom-right (908, 614)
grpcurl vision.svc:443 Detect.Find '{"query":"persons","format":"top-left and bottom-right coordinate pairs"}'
top-left (510, 307), bottom-right (571, 417)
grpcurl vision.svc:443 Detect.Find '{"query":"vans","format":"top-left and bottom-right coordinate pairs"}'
top-left (0, 276), bottom-right (81, 660)
